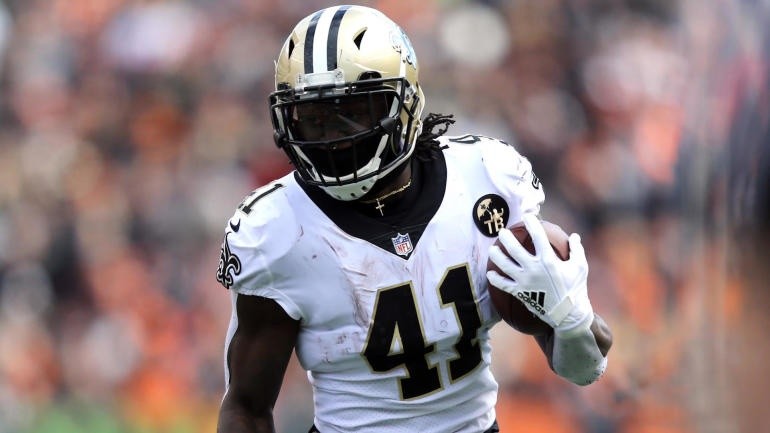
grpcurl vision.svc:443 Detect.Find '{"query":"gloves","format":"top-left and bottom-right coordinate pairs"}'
top-left (484, 213), bottom-right (593, 332)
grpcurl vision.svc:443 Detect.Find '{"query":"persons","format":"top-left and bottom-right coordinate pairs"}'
top-left (216, 5), bottom-right (612, 433)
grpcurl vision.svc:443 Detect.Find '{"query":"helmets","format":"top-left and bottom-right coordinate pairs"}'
top-left (267, 5), bottom-right (426, 202)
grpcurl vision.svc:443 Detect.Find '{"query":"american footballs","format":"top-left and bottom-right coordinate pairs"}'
top-left (485, 219), bottom-right (571, 335)
